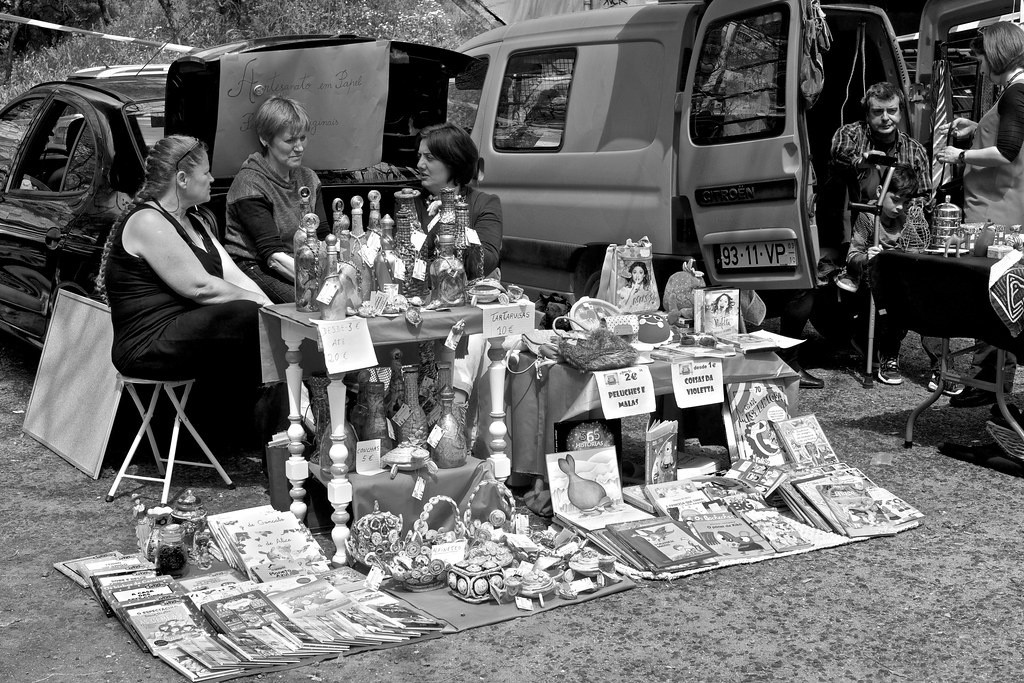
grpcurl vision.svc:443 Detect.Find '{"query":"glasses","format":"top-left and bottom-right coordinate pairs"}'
top-left (977, 25), bottom-right (987, 36)
top-left (176, 138), bottom-right (206, 171)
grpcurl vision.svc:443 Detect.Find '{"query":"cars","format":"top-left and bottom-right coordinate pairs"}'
top-left (0, 33), bottom-right (488, 349)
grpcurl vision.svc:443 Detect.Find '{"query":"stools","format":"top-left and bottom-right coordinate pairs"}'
top-left (105, 373), bottom-right (237, 508)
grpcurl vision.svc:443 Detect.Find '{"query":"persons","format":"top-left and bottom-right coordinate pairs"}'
top-left (781, 291), bottom-right (824, 388)
top-left (620, 262), bottom-right (655, 309)
top-left (226, 94), bottom-right (331, 303)
top-left (417, 123), bottom-right (502, 278)
top-left (846, 164), bottom-right (972, 396)
top-left (938, 21), bottom-right (1024, 407)
top-left (828, 82), bottom-right (937, 293)
top-left (711, 293), bottom-right (735, 326)
top-left (96, 134), bottom-right (281, 453)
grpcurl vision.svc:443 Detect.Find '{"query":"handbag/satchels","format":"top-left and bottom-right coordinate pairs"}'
top-left (552, 316), bottom-right (639, 371)
top-left (506, 330), bottom-right (567, 374)
top-left (935, 125), bottom-right (965, 208)
top-left (597, 236), bottom-right (660, 314)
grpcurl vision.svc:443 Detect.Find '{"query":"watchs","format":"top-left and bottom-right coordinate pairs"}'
top-left (959, 149), bottom-right (966, 164)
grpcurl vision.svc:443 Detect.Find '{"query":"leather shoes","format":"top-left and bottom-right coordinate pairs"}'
top-left (784, 360), bottom-right (823, 389)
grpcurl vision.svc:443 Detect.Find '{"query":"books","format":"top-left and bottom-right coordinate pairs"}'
top-left (53, 504), bottom-right (447, 683)
top-left (545, 379), bottom-right (925, 576)
top-left (651, 286), bottom-right (808, 363)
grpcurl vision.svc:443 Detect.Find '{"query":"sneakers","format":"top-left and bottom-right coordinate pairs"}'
top-left (833, 267), bottom-right (861, 293)
top-left (877, 349), bottom-right (902, 385)
top-left (851, 333), bottom-right (880, 368)
top-left (949, 386), bottom-right (996, 407)
top-left (928, 369), bottom-right (965, 396)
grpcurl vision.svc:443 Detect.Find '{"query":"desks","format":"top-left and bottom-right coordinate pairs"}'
top-left (867, 247), bottom-right (1024, 449)
top-left (258, 295), bottom-right (543, 568)
top-left (476, 326), bottom-right (799, 493)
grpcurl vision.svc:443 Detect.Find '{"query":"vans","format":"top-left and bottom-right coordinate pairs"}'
top-left (447, 0), bottom-right (1023, 306)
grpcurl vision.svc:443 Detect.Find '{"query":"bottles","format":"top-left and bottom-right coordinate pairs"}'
top-left (294, 186), bottom-right (485, 474)
top-left (145, 489), bottom-right (212, 580)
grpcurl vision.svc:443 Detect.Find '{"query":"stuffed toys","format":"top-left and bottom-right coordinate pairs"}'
top-left (630, 313), bottom-right (675, 351)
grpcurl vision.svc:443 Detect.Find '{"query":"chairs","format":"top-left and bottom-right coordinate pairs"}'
top-left (48, 118), bottom-right (85, 192)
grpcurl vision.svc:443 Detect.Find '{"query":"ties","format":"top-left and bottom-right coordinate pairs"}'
top-left (929, 59), bottom-right (955, 204)
